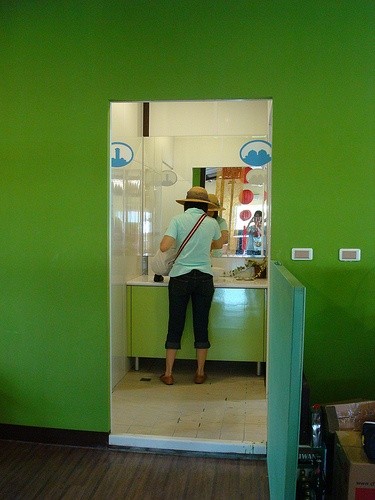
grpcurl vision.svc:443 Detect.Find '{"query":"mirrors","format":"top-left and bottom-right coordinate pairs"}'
top-left (193, 168), bottom-right (269, 258)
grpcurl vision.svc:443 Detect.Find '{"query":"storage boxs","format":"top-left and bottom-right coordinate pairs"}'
top-left (333, 428), bottom-right (375, 500)
top-left (321, 397), bottom-right (375, 434)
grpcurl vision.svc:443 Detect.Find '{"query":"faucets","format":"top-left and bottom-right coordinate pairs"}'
top-left (226, 244), bottom-right (230, 257)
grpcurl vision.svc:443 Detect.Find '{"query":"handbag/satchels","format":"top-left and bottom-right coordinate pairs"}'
top-left (151, 248), bottom-right (176, 276)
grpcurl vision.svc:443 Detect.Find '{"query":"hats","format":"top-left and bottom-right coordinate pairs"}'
top-left (207, 194), bottom-right (227, 210)
top-left (176, 186), bottom-right (218, 207)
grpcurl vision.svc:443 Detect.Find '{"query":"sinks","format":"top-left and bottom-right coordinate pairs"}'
top-left (212, 275), bottom-right (236, 285)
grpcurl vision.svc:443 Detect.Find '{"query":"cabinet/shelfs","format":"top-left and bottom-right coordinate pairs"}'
top-left (142, 100), bottom-right (271, 136)
top-left (126, 287), bottom-right (267, 363)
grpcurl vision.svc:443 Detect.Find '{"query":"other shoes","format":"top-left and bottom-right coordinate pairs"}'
top-left (193, 373), bottom-right (208, 384)
top-left (160, 375), bottom-right (174, 385)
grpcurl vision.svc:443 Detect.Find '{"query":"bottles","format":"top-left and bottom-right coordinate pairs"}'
top-left (296, 455), bottom-right (328, 500)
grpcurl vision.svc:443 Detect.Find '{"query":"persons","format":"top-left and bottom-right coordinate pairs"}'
top-left (246, 210), bottom-right (266, 255)
top-left (159, 186), bottom-right (223, 385)
top-left (206, 194), bottom-right (229, 257)
